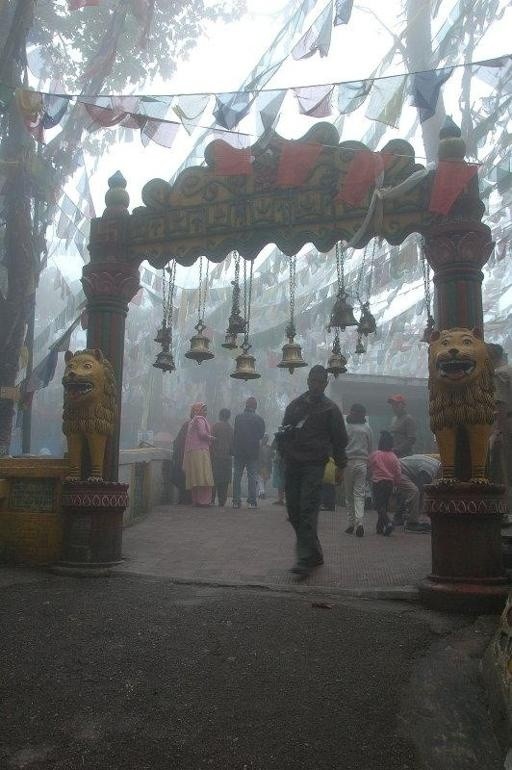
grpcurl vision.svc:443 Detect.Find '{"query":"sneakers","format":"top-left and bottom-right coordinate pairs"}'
top-left (272, 500), bottom-right (285, 506)
top-left (247, 502), bottom-right (259, 510)
top-left (287, 553), bottom-right (324, 575)
top-left (344, 525), bottom-right (365, 537)
top-left (377, 519), bottom-right (429, 536)
top-left (232, 501), bottom-right (241, 509)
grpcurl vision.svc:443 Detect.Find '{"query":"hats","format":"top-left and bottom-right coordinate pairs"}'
top-left (387, 394), bottom-right (407, 405)
top-left (245, 396), bottom-right (257, 408)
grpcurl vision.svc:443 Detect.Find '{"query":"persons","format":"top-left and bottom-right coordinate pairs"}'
top-left (343, 403), bottom-right (372, 536)
top-left (182, 402), bottom-right (216, 508)
top-left (270, 438), bottom-right (285, 507)
top-left (388, 394), bottom-right (416, 526)
top-left (258, 433), bottom-right (274, 501)
top-left (232, 396), bottom-right (265, 509)
top-left (270, 364), bottom-right (349, 577)
top-left (168, 407), bottom-right (192, 506)
top-left (321, 457), bottom-right (339, 512)
top-left (209, 408), bottom-right (234, 507)
top-left (367, 430), bottom-right (401, 537)
top-left (394, 454), bottom-right (441, 534)
